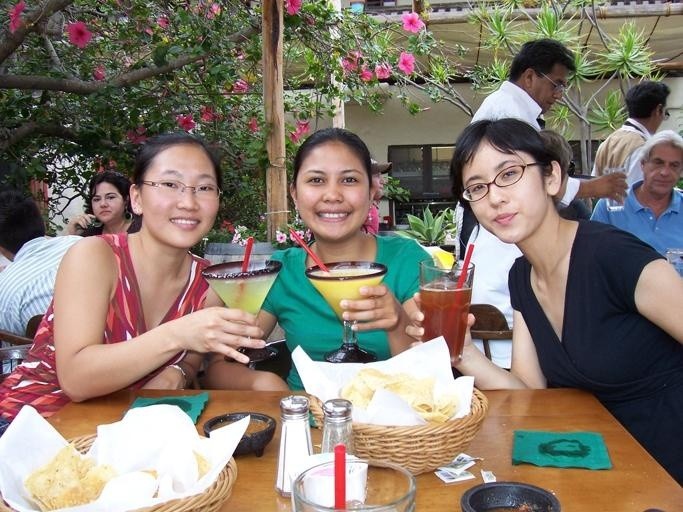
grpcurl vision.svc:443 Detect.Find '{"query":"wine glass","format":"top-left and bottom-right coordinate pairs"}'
top-left (306, 261), bottom-right (389, 366)
top-left (200, 260), bottom-right (283, 365)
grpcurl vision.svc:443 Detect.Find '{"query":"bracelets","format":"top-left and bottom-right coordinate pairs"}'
top-left (166, 363), bottom-right (190, 389)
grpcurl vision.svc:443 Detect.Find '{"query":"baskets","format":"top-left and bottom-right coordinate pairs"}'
top-left (0, 427), bottom-right (239, 512)
top-left (304, 372), bottom-right (491, 478)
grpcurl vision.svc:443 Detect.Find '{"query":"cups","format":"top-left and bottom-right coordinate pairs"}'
top-left (417, 256), bottom-right (476, 368)
top-left (290, 459), bottom-right (417, 511)
top-left (603, 166), bottom-right (627, 211)
top-left (663, 248), bottom-right (683, 278)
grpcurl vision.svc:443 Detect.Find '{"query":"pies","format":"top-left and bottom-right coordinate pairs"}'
top-left (341, 368), bottom-right (458, 423)
top-left (24, 442), bottom-right (211, 512)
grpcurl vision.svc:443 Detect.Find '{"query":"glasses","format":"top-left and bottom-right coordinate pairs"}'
top-left (137, 177), bottom-right (225, 201)
top-left (535, 70), bottom-right (569, 95)
top-left (460, 160), bottom-right (546, 204)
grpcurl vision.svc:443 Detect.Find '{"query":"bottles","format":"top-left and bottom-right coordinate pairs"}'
top-left (274, 396), bottom-right (358, 505)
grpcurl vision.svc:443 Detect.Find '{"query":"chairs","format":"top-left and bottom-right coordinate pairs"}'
top-left (460, 302), bottom-right (515, 371)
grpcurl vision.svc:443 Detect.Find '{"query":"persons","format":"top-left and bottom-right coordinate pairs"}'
top-left (361, 157), bottom-right (394, 233)
top-left (0, 133), bottom-right (265, 445)
top-left (590, 130), bottom-right (683, 275)
top-left (458, 38), bottom-right (629, 259)
top-left (205, 127), bottom-right (437, 392)
top-left (592, 83), bottom-right (671, 195)
top-left (461, 128), bottom-right (573, 371)
top-left (59, 171), bottom-right (141, 237)
top-left (405, 119), bottom-right (683, 487)
top-left (0, 189), bottom-right (83, 359)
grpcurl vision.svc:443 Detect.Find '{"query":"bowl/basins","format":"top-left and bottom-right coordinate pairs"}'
top-left (202, 411), bottom-right (277, 458)
top-left (460, 482), bottom-right (562, 511)
